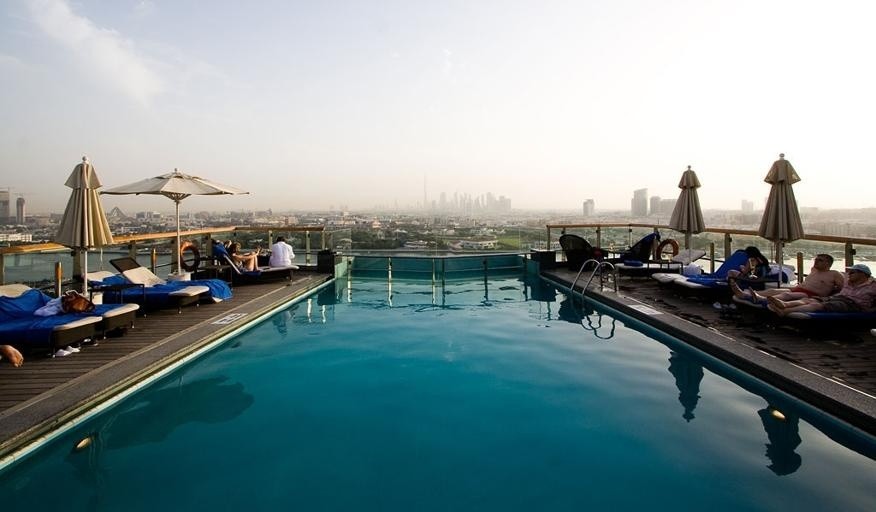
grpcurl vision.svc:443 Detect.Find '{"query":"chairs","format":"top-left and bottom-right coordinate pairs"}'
top-left (620, 233), bottom-right (657, 262)
top-left (732, 272), bottom-right (876, 343)
top-left (650, 250), bottom-right (797, 302)
top-left (613, 249), bottom-right (707, 284)
top-left (558, 234), bottom-right (608, 269)
top-left (215, 239), bottom-right (300, 286)
top-left (0, 257), bottom-right (232, 357)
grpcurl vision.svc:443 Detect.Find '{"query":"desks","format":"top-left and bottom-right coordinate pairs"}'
top-left (607, 249), bottom-right (633, 263)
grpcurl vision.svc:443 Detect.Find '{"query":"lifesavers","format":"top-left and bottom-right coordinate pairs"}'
top-left (657, 239), bottom-right (679, 260)
top-left (180, 242), bottom-right (200, 272)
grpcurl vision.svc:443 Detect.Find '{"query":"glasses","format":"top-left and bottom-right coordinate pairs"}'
top-left (848, 271), bottom-right (854, 275)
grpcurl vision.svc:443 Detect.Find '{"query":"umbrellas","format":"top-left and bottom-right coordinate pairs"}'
top-left (759, 153), bottom-right (804, 283)
top-left (54, 155), bottom-right (113, 295)
top-left (668, 165), bottom-right (705, 250)
top-left (98, 168), bottom-right (250, 274)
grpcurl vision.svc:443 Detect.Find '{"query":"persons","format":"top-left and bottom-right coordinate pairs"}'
top-left (730, 254), bottom-right (876, 316)
top-left (0, 345), bottom-right (24, 368)
top-left (269, 236), bottom-right (295, 267)
top-left (223, 240), bottom-right (261, 272)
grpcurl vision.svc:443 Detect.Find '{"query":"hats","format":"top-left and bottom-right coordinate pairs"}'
top-left (845, 263), bottom-right (872, 275)
